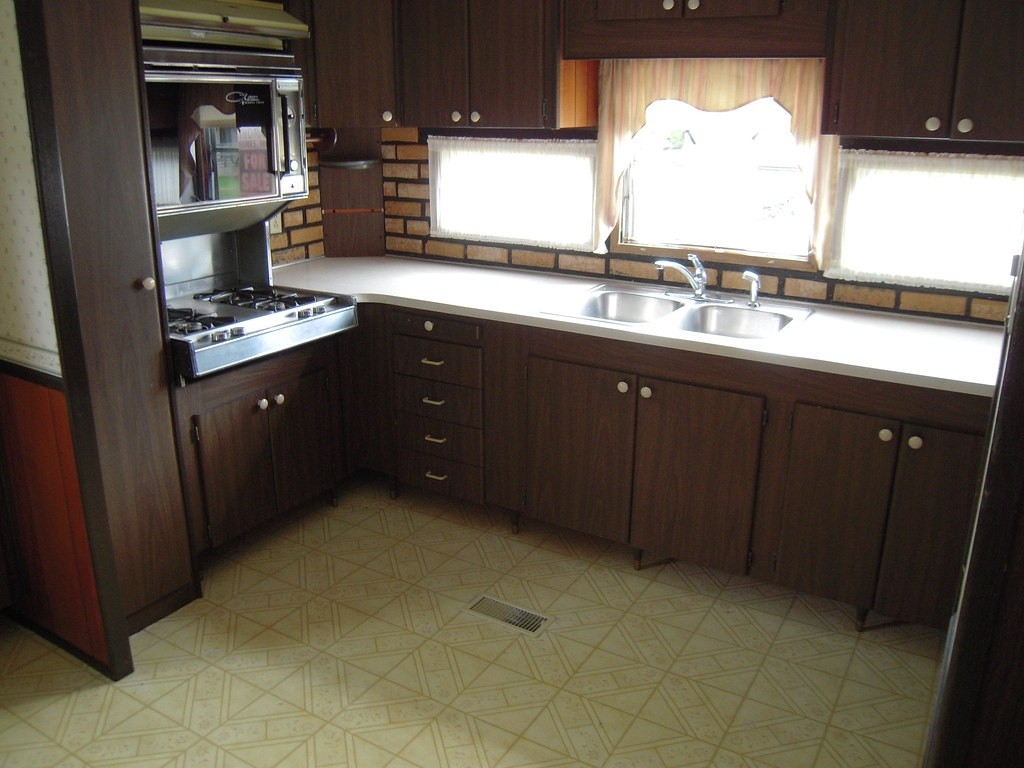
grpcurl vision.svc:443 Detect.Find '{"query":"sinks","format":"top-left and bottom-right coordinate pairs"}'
top-left (677, 299), bottom-right (821, 344)
top-left (540, 279), bottom-right (684, 330)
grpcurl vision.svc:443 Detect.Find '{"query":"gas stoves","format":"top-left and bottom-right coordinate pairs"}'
top-left (166, 285), bottom-right (358, 376)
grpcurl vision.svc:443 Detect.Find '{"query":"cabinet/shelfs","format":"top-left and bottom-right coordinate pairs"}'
top-left (168, 334), bottom-right (343, 582)
top-left (401, 0), bottom-right (597, 137)
top-left (820, 1), bottom-right (1023, 158)
top-left (340, 298), bottom-right (499, 503)
top-left (306, 1), bottom-right (397, 127)
top-left (762, 366), bottom-right (994, 633)
top-left (558, 0), bottom-right (833, 58)
top-left (500, 322), bottom-right (777, 579)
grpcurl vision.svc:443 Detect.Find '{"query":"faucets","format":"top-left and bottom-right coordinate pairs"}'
top-left (654, 259), bottom-right (703, 296)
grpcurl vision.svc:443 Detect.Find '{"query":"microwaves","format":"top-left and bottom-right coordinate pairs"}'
top-left (140, 46), bottom-right (308, 217)
